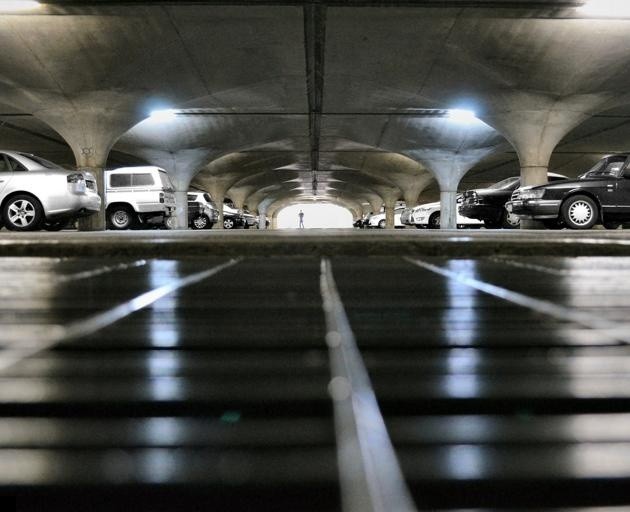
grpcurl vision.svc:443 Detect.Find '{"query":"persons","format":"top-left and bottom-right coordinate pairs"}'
top-left (365, 211), bottom-right (374, 228)
top-left (298, 209), bottom-right (304, 228)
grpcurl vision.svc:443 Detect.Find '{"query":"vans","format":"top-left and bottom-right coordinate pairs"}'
top-left (104, 165), bottom-right (176, 231)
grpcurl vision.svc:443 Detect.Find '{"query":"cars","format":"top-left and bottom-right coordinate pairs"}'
top-left (504, 152), bottom-right (630, 229)
top-left (188, 191), bottom-right (271, 229)
top-left (459, 171), bottom-right (570, 228)
top-left (0, 148), bottom-right (101, 232)
top-left (353, 201), bottom-right (409, 229)
top-left (410, 194), bottom-right (484, 229)
